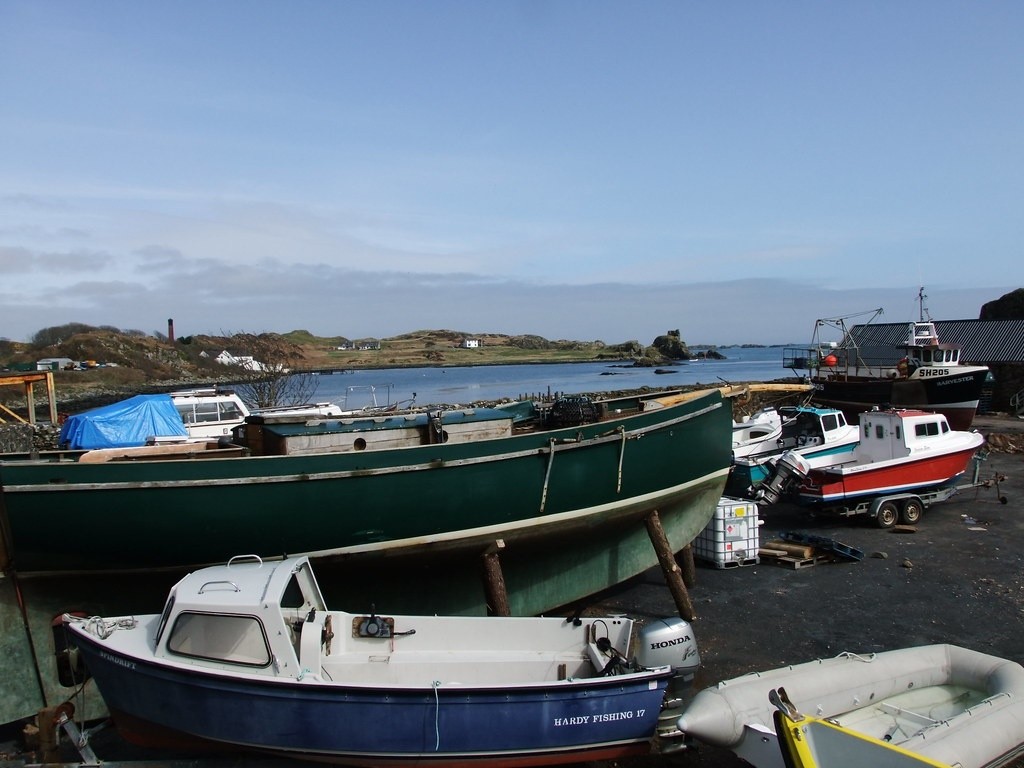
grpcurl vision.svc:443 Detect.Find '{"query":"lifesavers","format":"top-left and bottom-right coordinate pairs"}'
top-left (898, 358), bottom-right (908, 377)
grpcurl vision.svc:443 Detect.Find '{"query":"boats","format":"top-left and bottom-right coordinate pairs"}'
top-left (795, 405), bottom-right (984, 509)
top-left (62, 389), bottom-right (361, 454)
top-left (678, 645), bottom-right (1024, 768)
top-left (63, 553), bottom-right (700, 768)
top-left (729, 407), bottom-right (861, 491)
top-left (807, 288), bottom-right (989, 432)
top-left (732, 409), bottom-right (786, 449)
top-left (0, 385), bottom-right (730, 618)
